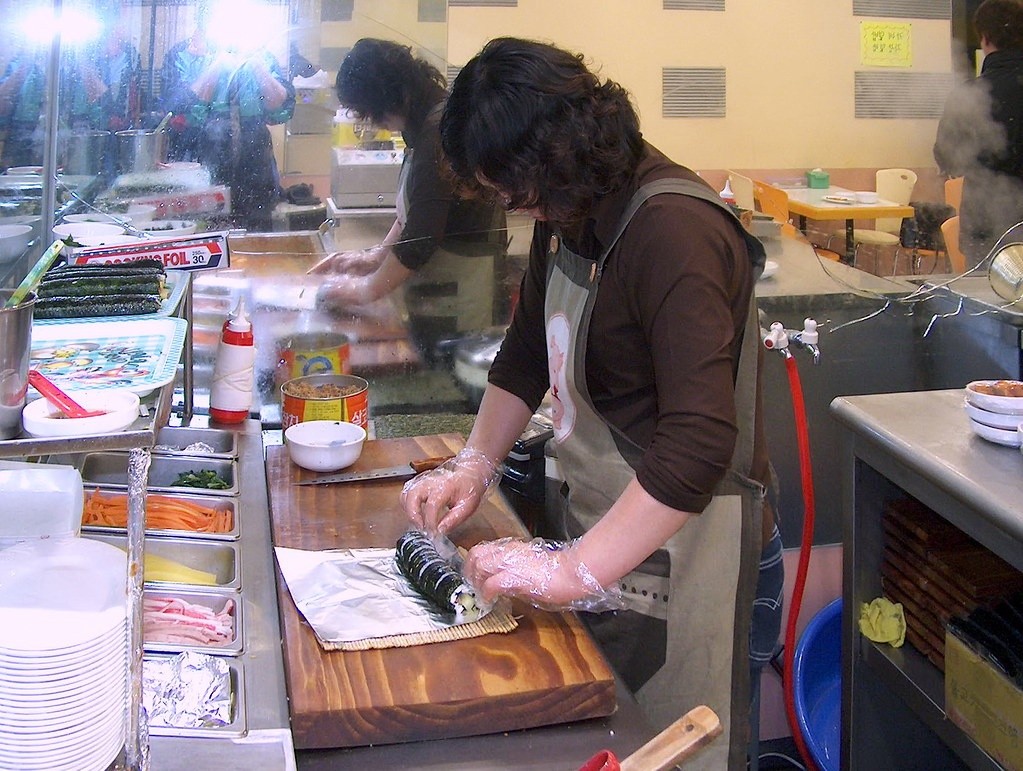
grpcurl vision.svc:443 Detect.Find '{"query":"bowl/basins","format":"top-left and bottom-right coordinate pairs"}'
top-left (52, 222), bottom-right (142, 260)
top-left (62, 204), bottom-right (197, 238)
top-left (0, 224), bottom-right (33, 263)
top-left (22, 389), bottom-right (141, 439)
top-left (855, 192), bottom-right (879, 203)
top-left (791, 597), bottom-right (843, 771)
top-left (284, 420), bottom-right (367, 473)
top-left (760, 261), bottom-right (778, 280)
top-left (964, 380), bottom-right (1023, 450)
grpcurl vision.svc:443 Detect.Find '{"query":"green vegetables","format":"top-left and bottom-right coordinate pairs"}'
top-left (169, 469), bottom-right (229, 490)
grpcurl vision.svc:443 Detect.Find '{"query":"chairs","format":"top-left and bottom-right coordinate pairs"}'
top-left (752, 178), bottom-right (840, 263)
top-left (941, 215), bottom-right (965, 275)
top-left (892, 177), bottom-right (965, 275)
top-left (827, 168), bottom-right (918, 275)
top-left (726, 169), bottom-right (754, 211)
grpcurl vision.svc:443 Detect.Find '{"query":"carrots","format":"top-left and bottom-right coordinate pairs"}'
top-left (83, 486), bottom-right (233, 533)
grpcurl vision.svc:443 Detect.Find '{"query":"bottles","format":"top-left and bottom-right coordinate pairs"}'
top-left (719, 180), bottom-right (736, 206)
top-left (209, 300), bottom-right (254, 425)
top-left (219, 295), bottom-right (252, 341)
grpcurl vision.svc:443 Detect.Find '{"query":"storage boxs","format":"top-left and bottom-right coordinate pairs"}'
top-left (945, 628), bottom-right (1023, 771)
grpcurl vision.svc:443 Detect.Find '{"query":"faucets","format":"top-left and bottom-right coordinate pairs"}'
top-left (794, 315), bottom-right (822, 368)
top-left (763, 319), bottom-right (797, 372)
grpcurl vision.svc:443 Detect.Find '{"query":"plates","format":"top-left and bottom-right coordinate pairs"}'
top-left (835, 192), bottom-right (854, 198)
top-left (823, 195), bottom-right (857, 204)
top-left (0, 536), bottom-right (126, 771)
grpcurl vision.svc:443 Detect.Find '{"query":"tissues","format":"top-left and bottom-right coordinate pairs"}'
top-left (805, 165), bottom-right (828, 189)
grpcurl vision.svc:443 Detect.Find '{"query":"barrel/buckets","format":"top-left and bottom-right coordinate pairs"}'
top-left (280, 372), bottom-right (369, 443)
top-left (275, 331), bottom-right (351, 407)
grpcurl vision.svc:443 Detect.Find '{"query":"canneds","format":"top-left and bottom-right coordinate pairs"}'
top-left (274, 330), bottom-right (370, 444)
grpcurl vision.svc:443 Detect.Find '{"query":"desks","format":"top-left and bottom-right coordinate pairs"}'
top-left (753, 186), bottom-right (914, 266)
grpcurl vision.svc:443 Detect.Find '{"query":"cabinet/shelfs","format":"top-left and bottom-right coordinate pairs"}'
top-left (827, 387), bottom-right (1023, 771)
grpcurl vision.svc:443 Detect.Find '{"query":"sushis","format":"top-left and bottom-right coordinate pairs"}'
top-left (395, 529), bottom-right (480, 616)
top-left (34, 259), bottom-right (167, 318)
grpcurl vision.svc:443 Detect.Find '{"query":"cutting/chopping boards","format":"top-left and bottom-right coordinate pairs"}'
top-left (252, 305), bottom-right (419, 374)
top-left (265, 432), bottom-right (618, 750)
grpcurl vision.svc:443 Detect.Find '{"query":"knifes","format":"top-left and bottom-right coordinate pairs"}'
top-left (290, 455), bottom-right (459, 486)
top-left (347, 330), bottom-right (408, 341)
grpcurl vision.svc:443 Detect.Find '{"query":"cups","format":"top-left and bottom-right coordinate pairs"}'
top-left (0, 288), bottom-right (39, 440)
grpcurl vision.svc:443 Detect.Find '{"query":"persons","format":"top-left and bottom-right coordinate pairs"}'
top-left (0, 0), bottom-right (296, 230)
top-left (933, 0), bottom-right (1023, 271)
top-left (314, 35), bottom-right (510, 368)
top-left (399, 36), bottom-right (784, 771)
